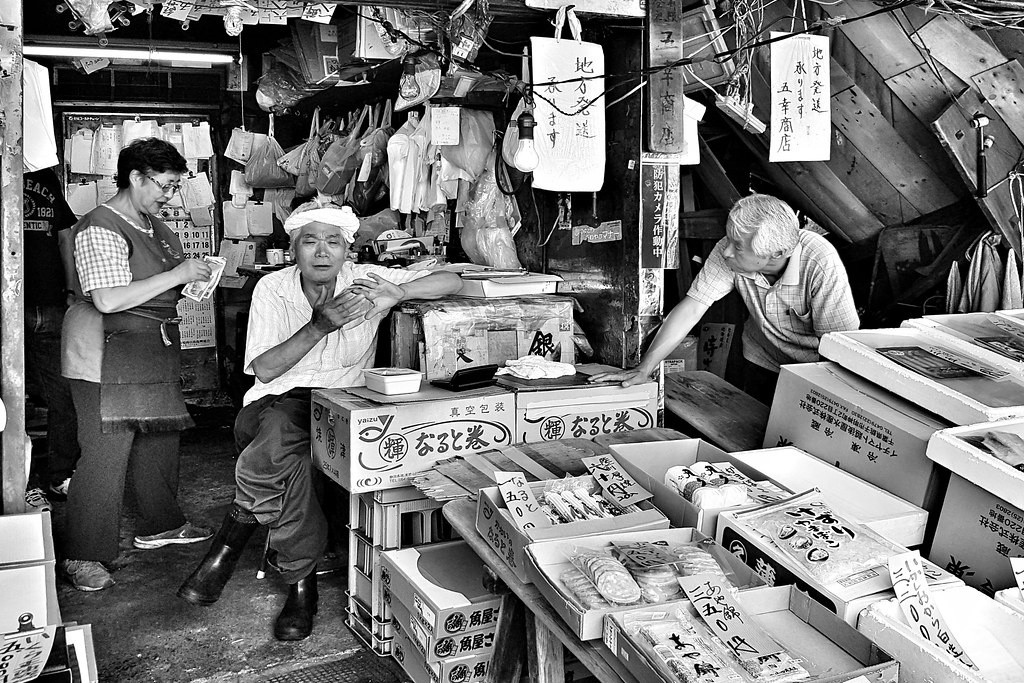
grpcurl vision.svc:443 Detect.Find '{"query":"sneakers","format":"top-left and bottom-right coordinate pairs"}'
top-left (24, 488), bottom-right (53, 513)
top-left (62, 559), bottom-right (116, 591)
top-left (50, 477), bottom-right (71, 495)
top-left (134, 521), bottom-right (214, 550)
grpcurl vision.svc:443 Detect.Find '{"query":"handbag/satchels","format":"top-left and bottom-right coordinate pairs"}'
top-left (244, 99), bottom-right (392, 198)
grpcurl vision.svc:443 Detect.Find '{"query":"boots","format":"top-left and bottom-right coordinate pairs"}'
top-left (176, 509), bottom-right (259, 607)
top-left (275, 564), bottom-right (319, 640)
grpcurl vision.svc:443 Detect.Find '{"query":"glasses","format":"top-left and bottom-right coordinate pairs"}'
top-left (143, 172), bottom-right (182, 194)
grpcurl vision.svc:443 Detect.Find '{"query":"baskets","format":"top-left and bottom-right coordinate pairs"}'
top-left (176, 293), bottom-right (216, 350)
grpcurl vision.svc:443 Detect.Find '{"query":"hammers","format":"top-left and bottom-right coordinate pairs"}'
top-left (972, 115), bottom-right (990, 198)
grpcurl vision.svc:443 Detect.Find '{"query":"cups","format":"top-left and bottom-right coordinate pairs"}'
top-left (266, 249), bottom-right (284, 265)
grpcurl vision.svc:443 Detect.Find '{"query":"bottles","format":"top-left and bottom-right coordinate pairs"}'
top-left (284, 250), bottom-right (291, 266)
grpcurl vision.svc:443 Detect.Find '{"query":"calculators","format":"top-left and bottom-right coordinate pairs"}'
top-left (430, 364), bottom-right (499, 392)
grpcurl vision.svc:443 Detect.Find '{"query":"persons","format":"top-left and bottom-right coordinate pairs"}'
top-left (586, 191), bottom-right (861, 409)
top-left (58, 135), bottom-right (216, 593)
top-left (23, 168), bottom-right (80, 513)
top-left (174, 192), bottom-right (467, 640)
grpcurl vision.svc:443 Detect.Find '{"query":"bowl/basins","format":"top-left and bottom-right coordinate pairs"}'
top-left (360, 368), bottom-right (425, 395)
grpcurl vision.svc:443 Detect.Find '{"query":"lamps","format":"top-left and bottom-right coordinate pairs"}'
top-left (22, 34), bottom-right (241, 63)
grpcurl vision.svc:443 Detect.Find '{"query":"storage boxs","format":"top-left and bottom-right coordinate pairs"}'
top-left (0, 267), bottom-right (1024, 683)
